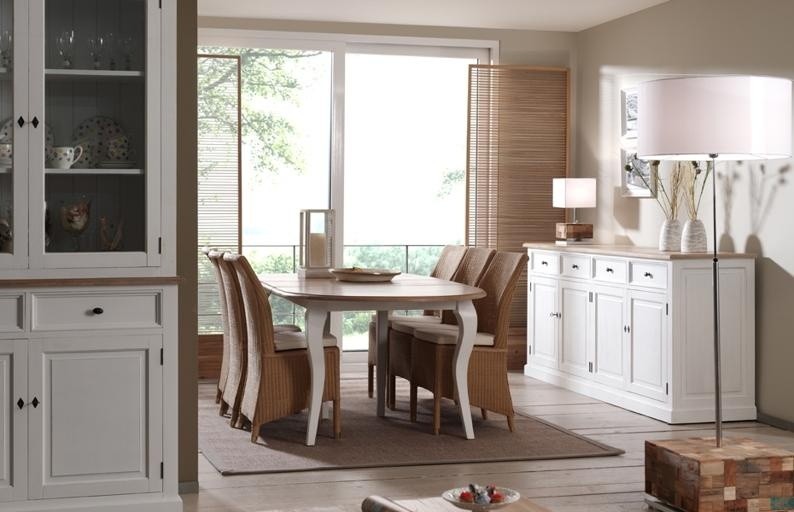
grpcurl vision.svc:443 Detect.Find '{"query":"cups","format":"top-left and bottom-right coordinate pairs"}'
top-left (48, 145), bottom-right (81, 168)
top-left (107, 134), bottom-right (137, 159)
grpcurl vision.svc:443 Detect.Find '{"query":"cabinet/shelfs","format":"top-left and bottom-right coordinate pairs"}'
top-left (0, 0), bottom-right (183, 511)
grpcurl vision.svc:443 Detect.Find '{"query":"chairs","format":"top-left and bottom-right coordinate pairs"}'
top-left (200, 246), bottom-right (223, 404)
top-left (409, 250), bottom-right (530, 437)
top-left (368, 244), bottom-right (469, 406)
top-left (388, 247), bottom-right (497, 422)
top-left (207, 250), bottom-right (247, 428)
top-left (223, 253), bottom-right (341, 444)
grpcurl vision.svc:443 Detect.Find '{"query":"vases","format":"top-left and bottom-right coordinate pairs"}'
top-left (679, 220), bottom-right (708, 252)
top-left (658, 218), bottom-right (679, 252)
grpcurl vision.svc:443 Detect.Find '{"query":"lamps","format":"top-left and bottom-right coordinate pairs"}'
top-left (636, 73), bottom-right (793, 511)
top-left (552, 177), bottom-right (597, 246)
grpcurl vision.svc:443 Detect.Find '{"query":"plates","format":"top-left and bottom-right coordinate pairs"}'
top-left (443, 484), bottom-right (522, 509)
top-left (81, 215), bottom-right (121, 248)
top-left (329, 268), bottom-right (402, 283)
top-left (72, 117), bottom-right (125, 169)
top-left (0, 118), bottom-right (54, 167)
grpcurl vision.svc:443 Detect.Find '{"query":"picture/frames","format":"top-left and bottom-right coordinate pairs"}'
top-left (617, 85), bottom-right (656, 198)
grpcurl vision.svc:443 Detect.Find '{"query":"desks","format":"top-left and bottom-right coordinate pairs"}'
top-left (257, 272), bottom-right (488, 447)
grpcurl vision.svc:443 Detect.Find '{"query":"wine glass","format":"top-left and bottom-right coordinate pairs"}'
top-left (0, 28), bottom-right (14, 72)
top-left (57, 31), bottom-right (138, 68)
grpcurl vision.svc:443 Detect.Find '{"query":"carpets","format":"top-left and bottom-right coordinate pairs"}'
top-left (196, 376), bottom-right (627, 476)
